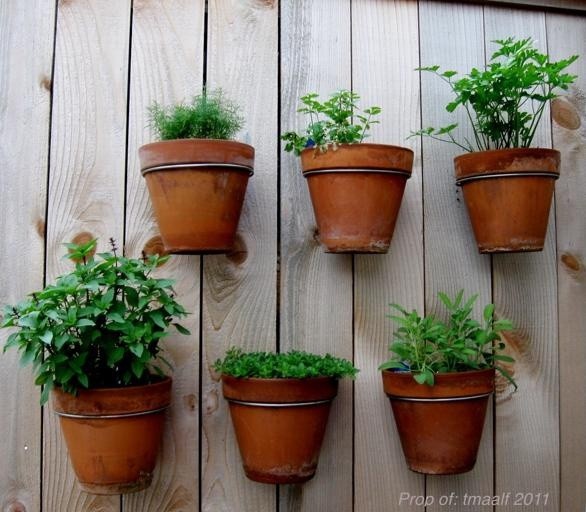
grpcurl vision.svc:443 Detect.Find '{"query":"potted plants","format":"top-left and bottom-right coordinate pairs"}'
top-left (139, 87), bottom-right (255, 255)
top-left (376, 289), bottom-right (516, 474)
top-left (408, 35), bottom-right (578, 252)
top-left (210, 346), bottom-right (359, 484)
top-left (1, 238), bottom-right (192, 497)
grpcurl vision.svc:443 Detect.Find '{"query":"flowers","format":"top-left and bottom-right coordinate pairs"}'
top-left (279, 90), bottom-right (413, 157)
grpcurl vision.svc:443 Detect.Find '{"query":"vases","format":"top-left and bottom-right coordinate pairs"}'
top-left (298, 143), bottom-right (415, 253)
